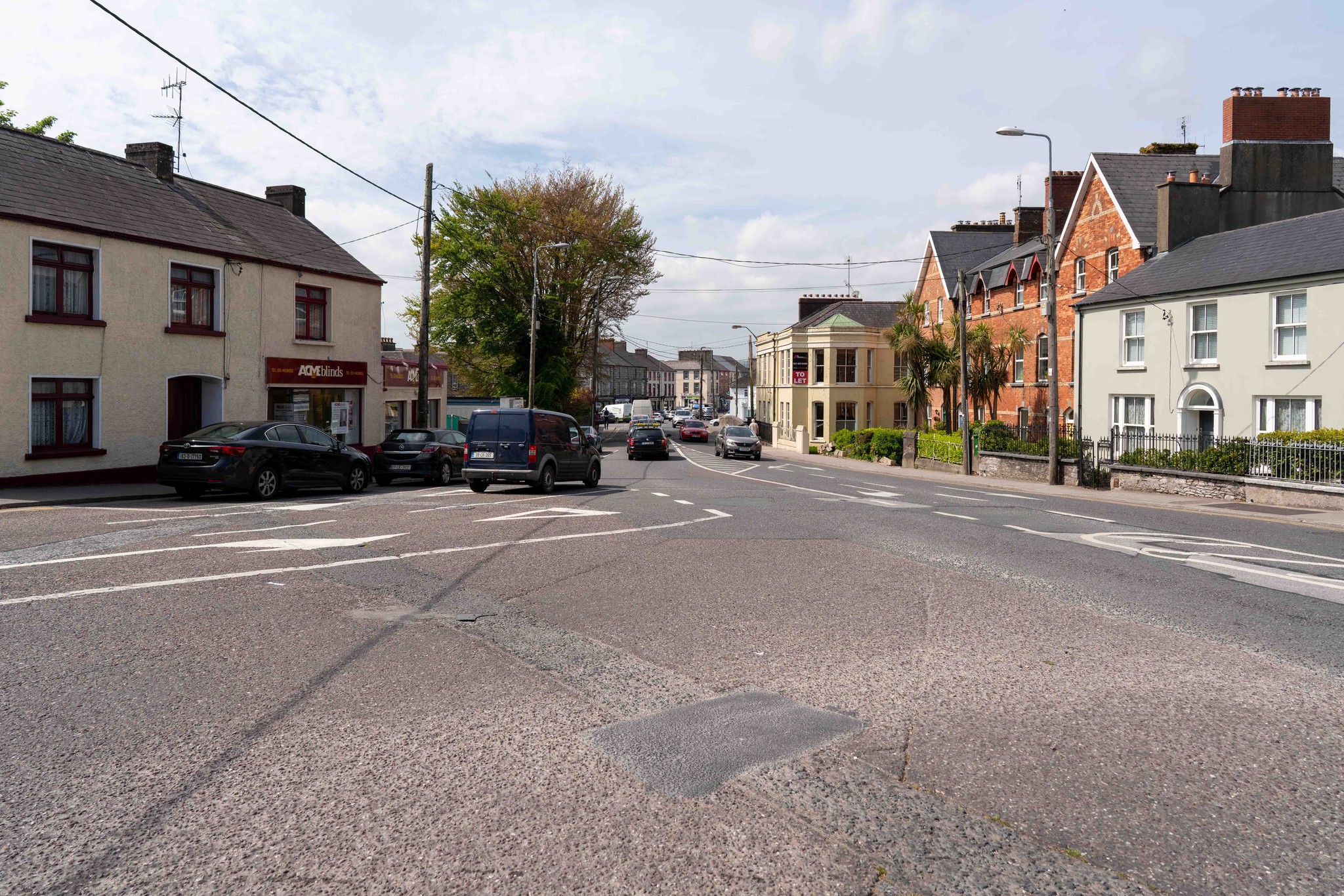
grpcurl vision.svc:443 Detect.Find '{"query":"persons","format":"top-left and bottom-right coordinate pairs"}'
top-left (594, 411), bottom-right (601, 433)
top-left (603, 407), bottom-right (610, 430)
top-left (749, 419), bottom-right (759, 437)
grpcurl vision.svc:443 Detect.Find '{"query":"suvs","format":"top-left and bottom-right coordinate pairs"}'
top-left (714, 425), bottom-right (762, 461)
top-left (671, 409), bottom-right (695, 428)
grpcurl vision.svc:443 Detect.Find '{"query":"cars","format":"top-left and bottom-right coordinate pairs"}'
top-left (709, 415), bottom-right (727, 426)
top-left (726, 410), bottom-right (730, 415)
top-left (627, 423), bottom-right (672, 460)
top-left (624, 404), bottom-right (714, 453)
top-left (155, 420), bottom-right (373, 500)
top-left (679, 419), bottom-right (709, 443)
top-left (703, 410), bottom-right (718, 421)
top-left (570, 425), bottom-right (603, 452)
top-left (371, 428), bottom-right (468, 486)
top-left (598, 412), bottom-right (615, 424)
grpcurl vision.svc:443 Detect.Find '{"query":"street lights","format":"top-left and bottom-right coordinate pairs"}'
top-left (701, 346), bottom-right (715, 419)
top-left (658, 360), bottom-right (669, 409)
top-left (591, 275), bottom-right (622, 426)
top-left (994, 126), bottom-right (1059, 486)
top-left (528, 242), bottom-right (570, 409)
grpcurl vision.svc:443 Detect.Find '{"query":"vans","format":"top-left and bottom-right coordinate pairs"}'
top-left (462, 408), bottom-right (601, 494)
top-left (602, 403), bottom-right (632, 423)
top-left (630, 400), bottom-right (653, 419)
